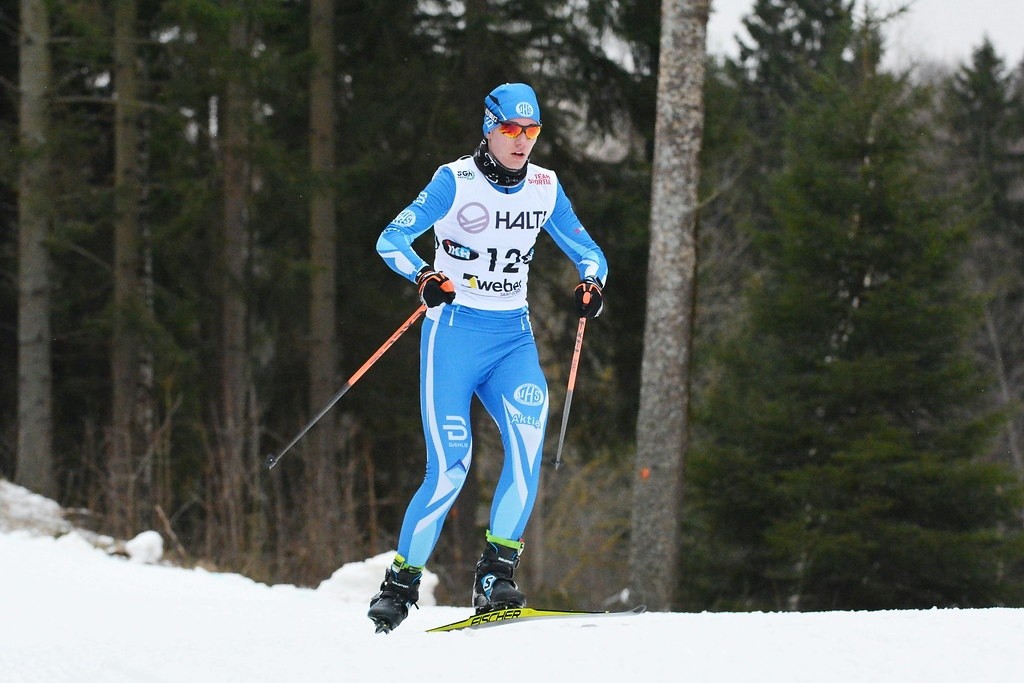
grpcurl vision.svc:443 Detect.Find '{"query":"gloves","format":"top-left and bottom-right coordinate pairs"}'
top-left (415, 266), bottom-right (456, 308)
top-left (572, 275), bottom-right (604, 318)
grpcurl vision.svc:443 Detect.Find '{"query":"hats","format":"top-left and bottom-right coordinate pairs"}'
top-left (482, 83), bottom-right (540, 138)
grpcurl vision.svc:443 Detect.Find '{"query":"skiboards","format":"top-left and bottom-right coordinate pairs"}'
top-left (374, 602), bottom-right (649, 637)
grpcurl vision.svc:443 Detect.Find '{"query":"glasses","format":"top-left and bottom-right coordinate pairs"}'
top-left (497, 120), bottom-right (542, 139)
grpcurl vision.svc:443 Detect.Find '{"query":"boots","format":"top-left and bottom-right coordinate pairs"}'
top-left (472, 529), bottom-right (527, 616)
top-left (367, 553), bottom-right (424, 633)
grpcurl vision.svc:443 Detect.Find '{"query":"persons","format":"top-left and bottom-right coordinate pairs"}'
top-left (367, 83), bottom-right (608, 634)
